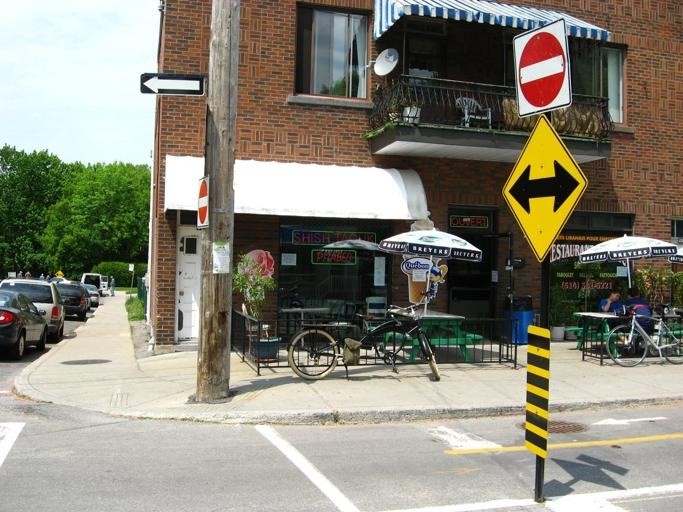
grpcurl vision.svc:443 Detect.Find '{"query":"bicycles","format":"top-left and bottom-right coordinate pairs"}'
top-left (286, 302), bottom-right (441, 380)
top-left (607, 305), bottom-right (683, 366)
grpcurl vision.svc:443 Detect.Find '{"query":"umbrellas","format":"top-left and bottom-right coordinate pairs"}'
top-left (578, 233), bottom-right (679, 289)
top-left (378, 227), bottom-right (483, 315)
top-left (669, 247), bottom-right (683, 265)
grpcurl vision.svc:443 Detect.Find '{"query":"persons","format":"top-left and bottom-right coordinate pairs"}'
top-left (46, 274), bottom-right (50, 280)
top-left (17, 270), bottom-right (23, 278)
top-left (109, 275), bottom-right (116, 296)
top-left (25, 271), bottom-right (31, 279)
top-left (598, 290), bottom-right (632, 353)
top-left (39, 273), bottom-right (44, 279)
top-left (620, 286), bottom-right (654, 353)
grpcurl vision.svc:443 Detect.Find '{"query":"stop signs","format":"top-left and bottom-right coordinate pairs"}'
top-left (512, 18), bottom-right (572, 119)
top-left (197, 176), bottom-right (210, 229)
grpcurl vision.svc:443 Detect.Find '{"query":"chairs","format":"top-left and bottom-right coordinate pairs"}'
top-left (241, 303), bottom-right (269, 354)
top-left (455, 97), bottom-right (491, 129)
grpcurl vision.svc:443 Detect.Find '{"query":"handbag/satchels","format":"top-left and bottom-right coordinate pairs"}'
top-left (342, 338), bottom-right (363, 366)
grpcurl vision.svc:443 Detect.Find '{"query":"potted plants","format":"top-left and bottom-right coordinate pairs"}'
top-left (403, 86), bottom-right (422, 124)
top-left (550, 283), bottom-right (564, 341)
top-left (565, 303), bottom-right (577, 340)
top-left (389, 96), bottom-right (403, 123)
top-left (231, 254), bottom-right (279, 360)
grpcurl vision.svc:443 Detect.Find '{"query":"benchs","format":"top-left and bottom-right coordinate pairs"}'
top-left (565, 327), bottom-right (593, 332)
top-left (438, 325), bottom-right (483, 345)
top-left (597, 329), bottom-right (683, 339)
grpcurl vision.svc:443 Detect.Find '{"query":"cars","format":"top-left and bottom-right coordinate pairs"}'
top-left (0, 277), bottom-right (100, 360)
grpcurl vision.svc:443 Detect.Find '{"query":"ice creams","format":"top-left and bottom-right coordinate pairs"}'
top-left (402, 217), bottom-right (438, 305)
top-left (238, 249), bottom-right (274, 301)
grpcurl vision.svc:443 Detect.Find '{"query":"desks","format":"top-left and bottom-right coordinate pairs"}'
top-left (574, 312), bottom-right (681, 359)
top-left (280, 308), bottom-right (329, 350)
top-left (387, 308), bottom-right (473, 363)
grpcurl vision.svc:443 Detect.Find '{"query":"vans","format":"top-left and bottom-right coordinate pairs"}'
top-left (81, 273), bottom-right (108, 297)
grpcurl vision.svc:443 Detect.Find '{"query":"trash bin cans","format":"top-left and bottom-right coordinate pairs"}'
top-left (505, 295), bottom-right (534, 344)
